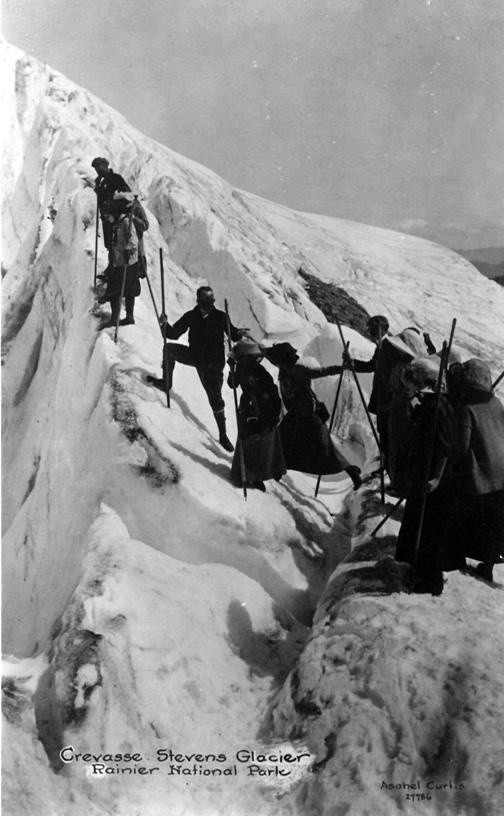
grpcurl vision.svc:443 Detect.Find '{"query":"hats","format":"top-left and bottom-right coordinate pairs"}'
top-left (401, 358), bottom-right (447, 395)
top-left (266, 343), bottom-right (299, 364)
top-left (237, 342), bottom-right (262, 355)
top-left (451, 358), bottom-right (492, 394)
top-left (92, 157), bottom-right (110, 167)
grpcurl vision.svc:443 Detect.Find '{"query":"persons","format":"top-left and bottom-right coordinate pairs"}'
top-left (346, 314), bottom-right (503, 597)
top-left (91, 150), bottom-right (130, 279)
top-left (146, 285), bottom-right (248, 452)
top-left (97, 198), bottom-right (135, 330)
top-left (225, 340), bottom-right (287, 492)
top-left (115, 191), bottom-right (149, 299)
top-left (257, 343), bottom-right (362, 492)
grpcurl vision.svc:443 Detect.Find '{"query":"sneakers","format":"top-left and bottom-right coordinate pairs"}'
top-left (470, 562), bottom-right (494, 583)
top-left (346, 465), bottom-right (363, 491)
top-left (219, 433), bottom-right (234, 452)
top-left (412, 572), bottom-right (444, 597)
top-left (371, 466), bottom-right (408, 501)
top-left (96, 273), bottom-right (115, 304)
top-left (103, 317), bottom-right (135, 328)
top-left (147, 374), bottom-right (169, 394)
top-left (234, 479), bottom-right (266, 492)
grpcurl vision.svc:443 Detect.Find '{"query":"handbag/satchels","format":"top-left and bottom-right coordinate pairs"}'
top-left (315, 401), bottom-right (330, 424)
